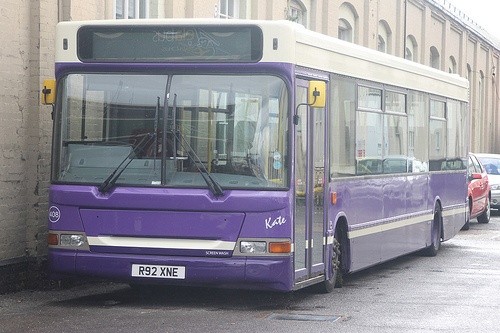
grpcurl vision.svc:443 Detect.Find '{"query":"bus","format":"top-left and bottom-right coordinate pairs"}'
top-left (41, 18), bottom-right (470, 297)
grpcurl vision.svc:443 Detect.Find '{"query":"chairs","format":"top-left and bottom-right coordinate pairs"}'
top-left (211, 158), bottom-right (227, 173)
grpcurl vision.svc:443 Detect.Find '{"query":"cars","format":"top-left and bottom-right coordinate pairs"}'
top-left (453, 153), bottom-right (491, 230)
top-left (472, 154), bottom-right (500, 216)
